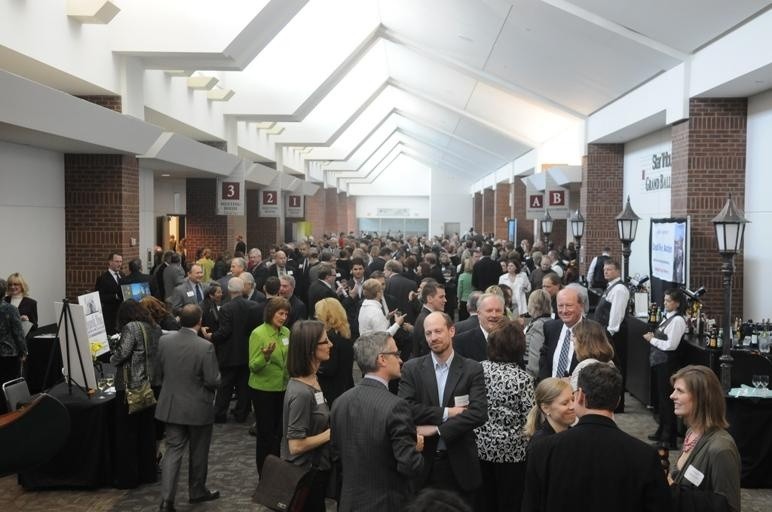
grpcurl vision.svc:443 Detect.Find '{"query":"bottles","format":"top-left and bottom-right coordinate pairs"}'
top-left (703, 324), bottom-right (723, 349)
top-left (647, 302), bottom-right (661, 324)
top-left (731, 318), bottom-right (772, 348)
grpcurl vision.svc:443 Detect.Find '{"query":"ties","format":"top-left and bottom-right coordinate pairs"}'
top-left (556, 329), bottom-right (572, 379)
top-left (116, 273), bottom-right (120, 282)
top-left (195, 285), bottom-right (203, 303)
top-left (439, 367), bottom-right (447, 404)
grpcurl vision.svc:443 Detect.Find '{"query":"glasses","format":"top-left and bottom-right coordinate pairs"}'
top-left (314, 337), bottom-right (328, 344)
top-left (378, 350), bottom-right (401, 358)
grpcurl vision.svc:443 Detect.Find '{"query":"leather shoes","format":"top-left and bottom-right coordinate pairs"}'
top-left (187, 490), bottom-right (220, 504)
top-left (159, 500), bottom-right (177, 512)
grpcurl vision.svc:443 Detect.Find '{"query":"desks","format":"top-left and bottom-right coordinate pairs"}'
top-left (22, 360), bottom-right (158, 489)
top-left (29, 333), bottom-right (67, 396)
top-left (584, 286), bottom-right (649, 413)
top-left (650, 322), bottom-right (771, 491)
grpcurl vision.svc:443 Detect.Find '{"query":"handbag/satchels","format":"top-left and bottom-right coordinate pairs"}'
top-left (126, 382), bottom-right (158, 414)
top-left (250, 453), bottom-right (312, 512)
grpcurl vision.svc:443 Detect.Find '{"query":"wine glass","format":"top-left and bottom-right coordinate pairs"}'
top-left (97, 373), bottom-right (115, 399)
top-left (752, 374), bottom-right (770, 396)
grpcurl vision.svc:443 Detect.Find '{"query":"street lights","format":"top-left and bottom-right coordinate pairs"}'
top-left (569, 205), bottom-right (590, 284)
top-left (613, 193), bottom-right (642, 283)
top-left (704, 189), bottom-right (754, 395)
top-left (540, 207), bottom-right (555, 256)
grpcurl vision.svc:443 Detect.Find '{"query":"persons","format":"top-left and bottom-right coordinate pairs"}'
top-left (95, 227), bottom-right (739, 511)
top-left (5, 273), bottom-right (39, 330)
top-left (1, 279), bottom-right (29, 410)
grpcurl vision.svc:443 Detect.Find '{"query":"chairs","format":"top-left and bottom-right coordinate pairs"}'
top-left (1, 377), bottom-right (33, 412)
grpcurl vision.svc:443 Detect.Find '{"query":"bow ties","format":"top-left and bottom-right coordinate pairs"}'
top-left (662, 314), bottom-right (667, 320)
